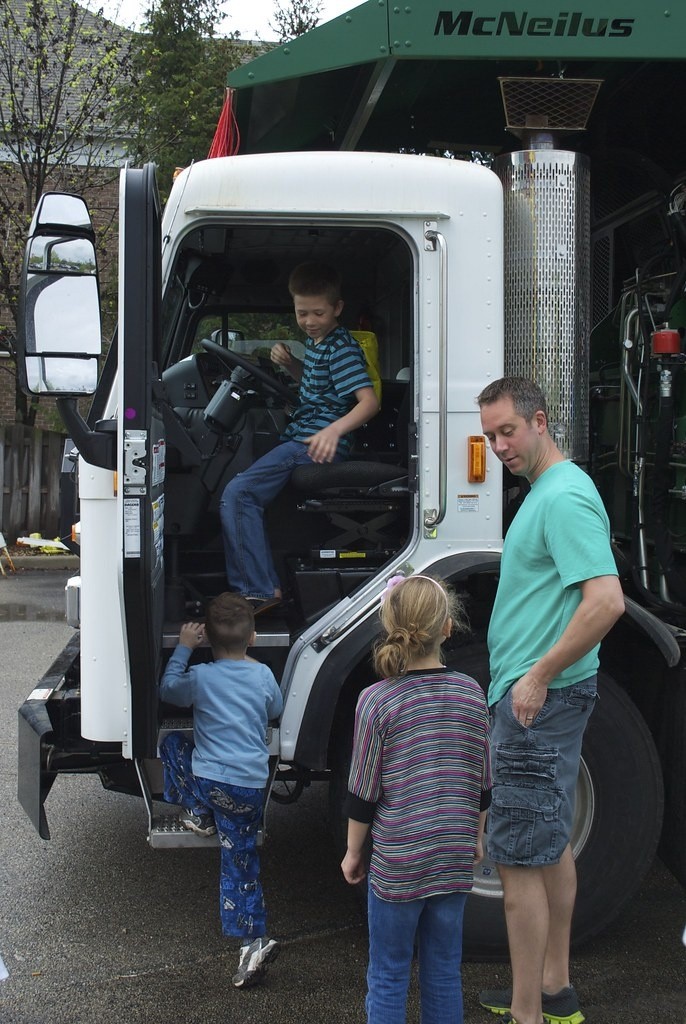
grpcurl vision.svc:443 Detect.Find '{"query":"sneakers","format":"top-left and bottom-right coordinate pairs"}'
top-left (178, 808), bottom-right (216, 836)
top-left (232, 935), bottom-right (280, 988)
top-left (478, 987), bottom-right (585, 1024)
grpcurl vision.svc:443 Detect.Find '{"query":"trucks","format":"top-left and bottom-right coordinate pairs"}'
top-left (12, 0), bottom-right (686, 962)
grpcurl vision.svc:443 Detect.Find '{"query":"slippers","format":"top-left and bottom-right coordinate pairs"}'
top-left (245, 587), bottom-right (282, 616)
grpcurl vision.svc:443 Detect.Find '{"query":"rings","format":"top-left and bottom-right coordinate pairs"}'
top-left (527, 717), bottom-right (533, 719)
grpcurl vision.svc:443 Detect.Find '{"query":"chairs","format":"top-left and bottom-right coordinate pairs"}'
top-left (292, 388), bottom-right (409, 560)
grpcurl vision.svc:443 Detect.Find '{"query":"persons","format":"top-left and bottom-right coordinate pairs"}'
top-left (158, 591), bottom-right (284, 988)
top-left (479, 377), bottom-right (625, 1024)
top-left (340, 575), bottom-right (491, 1024)
top-left (219, 262), bottom-right (379, 613)
